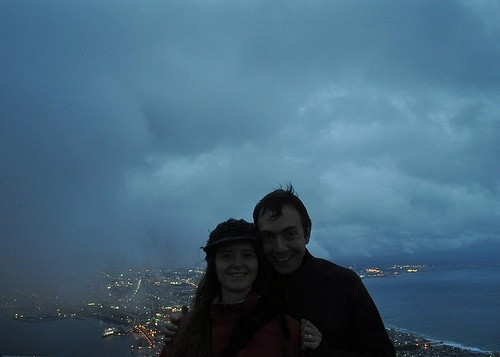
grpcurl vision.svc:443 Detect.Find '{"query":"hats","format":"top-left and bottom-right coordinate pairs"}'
top-left (204, 219), bottom-right (259, 252)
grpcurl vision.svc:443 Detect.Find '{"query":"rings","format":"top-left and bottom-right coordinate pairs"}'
top-left (308, 333), bottom-right (312, 342)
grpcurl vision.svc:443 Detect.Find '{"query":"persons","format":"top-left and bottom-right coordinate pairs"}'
top-left (158, 219), bottom-right (315, 357)
top-left (162, 185), bottom-right (397, 357)
